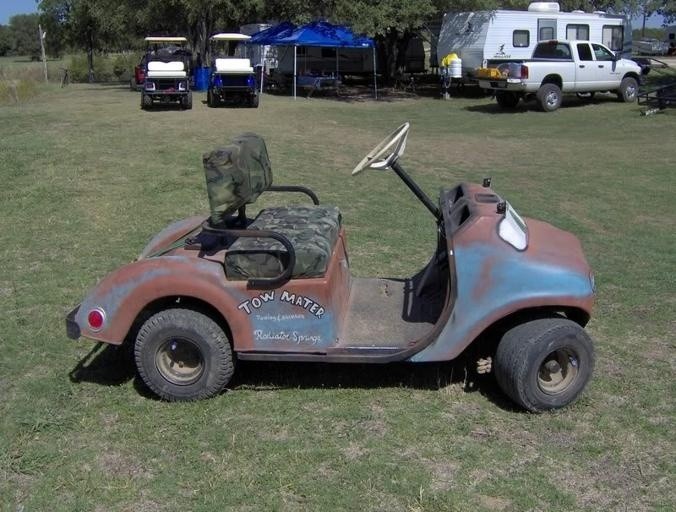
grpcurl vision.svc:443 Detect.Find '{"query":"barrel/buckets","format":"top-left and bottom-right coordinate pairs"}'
top-left (440, 65), bottom-right (448, 75)
top-left (448, 59), bottom-right (462, 78)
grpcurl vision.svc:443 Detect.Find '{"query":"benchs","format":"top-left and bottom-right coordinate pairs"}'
top-left (215, 56), bottom-right (254, 74)
top-left (148, 60), bottom-right (186, 77)
top-left (202, 128), bottom-right (344, 282)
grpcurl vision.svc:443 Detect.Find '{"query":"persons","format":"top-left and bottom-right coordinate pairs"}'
top-left (303, 64), bottom-right (328, 100)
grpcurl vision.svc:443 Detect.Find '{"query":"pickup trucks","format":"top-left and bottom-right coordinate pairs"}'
top-left (478, 40), bottom-right (642, 112)
